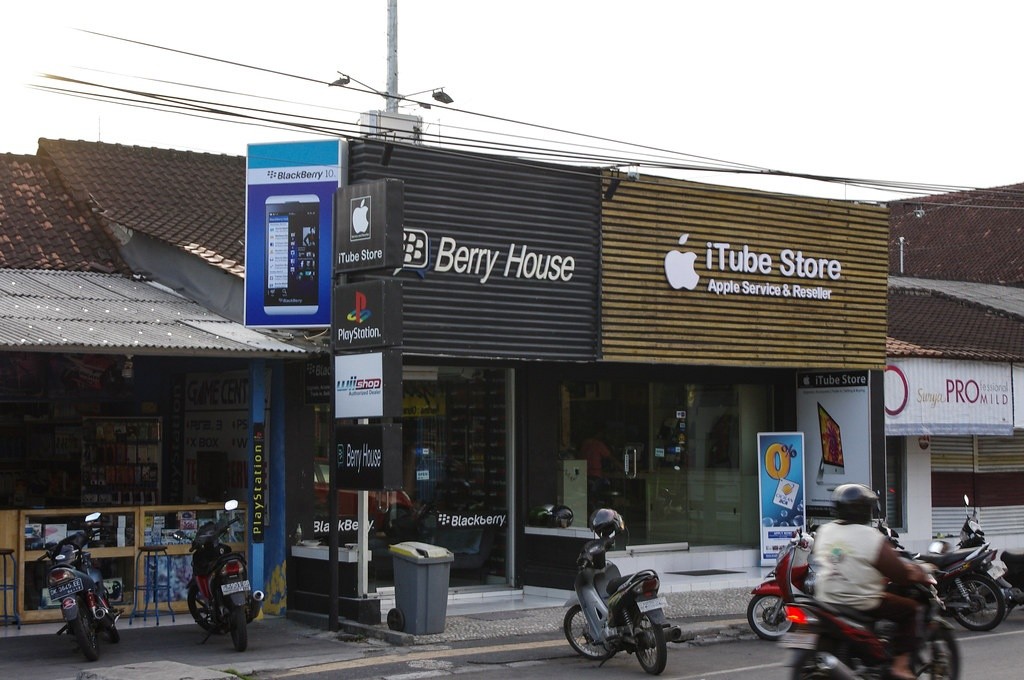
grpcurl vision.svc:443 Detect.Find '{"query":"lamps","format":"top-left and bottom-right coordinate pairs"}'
top-left (327, 71), bottom-right (385, 97)
top-left (914, 210), bottom-right (924, 220)
top-left (402, 87), bottom-right (454, 105)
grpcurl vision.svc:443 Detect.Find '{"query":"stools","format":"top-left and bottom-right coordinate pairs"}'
top-left (128, 545), bottom-right (175, 626)
top-left (594, 488), bottom-right (622, 510)
top-left (0, 548), bottom-right (22, 630)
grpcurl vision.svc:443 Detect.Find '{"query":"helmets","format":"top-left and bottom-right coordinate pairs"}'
top-left (536, 503), bottom-right (554, 528)
top-left (840, 486), bottom-right (879, 527)
top-left (588, 508), bottom-right (621, 536)
top-left (552, 505), bottom-right (574, 528)
top-left (832, 481), bottom-right (856, 501)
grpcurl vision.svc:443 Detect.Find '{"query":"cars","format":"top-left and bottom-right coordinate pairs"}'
top-left (311, 457), bottom-right (415, 544)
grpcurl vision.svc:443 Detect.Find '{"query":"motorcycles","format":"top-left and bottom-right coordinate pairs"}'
top-left (747, 527), bottom-right (819, 642)
top-left (173, 500), bottom-right (264, 652)
top-left (562, 509), bottom-right (682, 675)
top-left (36, 512), bottom-right (120, 662)
top-left (954, 493), bottom-right (1024, 624)
top-left (776, 567), bottom-right (962, 680)
top-left (874, 490), bottom-right (1005, 632)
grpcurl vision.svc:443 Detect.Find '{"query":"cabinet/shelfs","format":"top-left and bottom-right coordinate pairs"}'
top-left (80, 415), bottom-right (163, 506)
top-left (0, 503), bottom-right (248, 622)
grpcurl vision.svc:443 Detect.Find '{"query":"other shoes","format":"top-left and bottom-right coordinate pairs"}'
top-left (891, 664), bottom-right (916, 680)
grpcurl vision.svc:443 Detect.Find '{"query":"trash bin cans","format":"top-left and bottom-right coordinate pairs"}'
top-left (389, 541), bottom-right (454, 636)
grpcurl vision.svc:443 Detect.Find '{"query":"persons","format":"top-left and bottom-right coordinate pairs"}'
top-left (812, 488), bottom-right (938, 680)
top-left (579, 422), bottom-right (624, 513)
